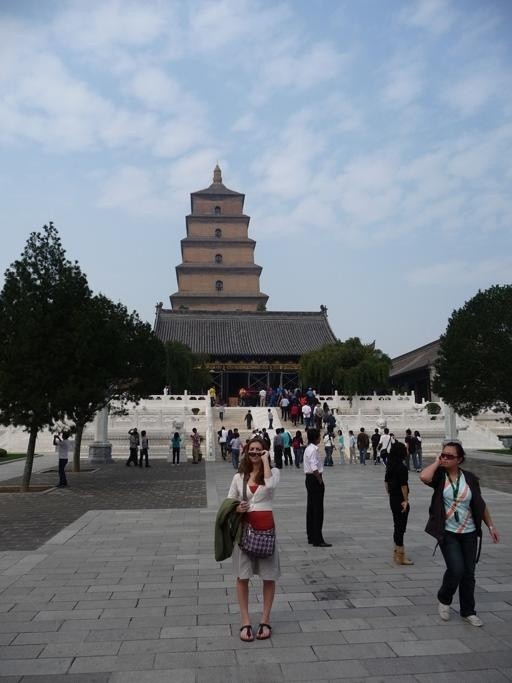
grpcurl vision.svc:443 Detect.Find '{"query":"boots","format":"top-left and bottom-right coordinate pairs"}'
top-left (393, 542), bottom-right (414, 565)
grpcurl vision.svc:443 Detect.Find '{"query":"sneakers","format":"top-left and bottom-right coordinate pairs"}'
top-left (437, 602), bottom-right (451, 621)
top-left (461, 614), bottom-right (484, 627)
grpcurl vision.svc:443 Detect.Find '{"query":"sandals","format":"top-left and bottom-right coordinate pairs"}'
top-left (256, 623), bottom-right (272, 640)
top-left (240, 625), bottom-right (254, 642)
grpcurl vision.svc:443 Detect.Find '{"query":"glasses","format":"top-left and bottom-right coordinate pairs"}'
top-left (441, 453), bottom-right (458, 460)
top-left (247, 451), bottom-right (265, 457)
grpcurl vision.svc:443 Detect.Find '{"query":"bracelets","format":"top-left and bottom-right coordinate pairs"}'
top-left (489, 525), bottom-right (494, 529)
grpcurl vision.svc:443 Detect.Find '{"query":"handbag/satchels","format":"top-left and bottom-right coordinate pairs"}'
top-left (380, 448), bottom-right (388, 458)
top-left (288, 438), bottom-right (293, 446)
top-left (237, 522), bottom-right (275, 556)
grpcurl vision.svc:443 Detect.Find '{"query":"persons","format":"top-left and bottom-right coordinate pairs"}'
top-left (383, 440), bottom-right (414, 565)
top-left (124, 382), bottom-right (423, 475)
top-left (418, 439), bottom-right (500, 627)
top-left (53, 430), bottom-right (70, 487)
top-left (227, 437), bottom-right (281, 641)
top-left (304, 428), bottom-right (333, 546)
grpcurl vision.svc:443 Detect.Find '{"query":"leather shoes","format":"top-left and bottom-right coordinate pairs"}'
top-left (308, 538), bottom-right (332, 548)
